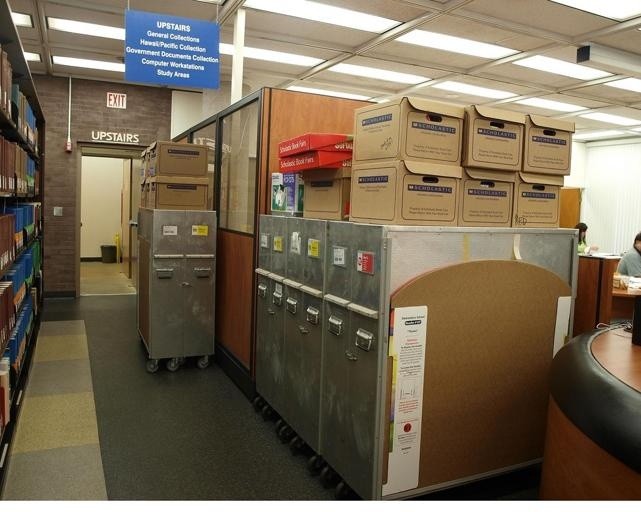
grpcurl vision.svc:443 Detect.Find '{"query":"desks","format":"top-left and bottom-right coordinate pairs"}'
top-left (548, 251), bottom-right (641, 473)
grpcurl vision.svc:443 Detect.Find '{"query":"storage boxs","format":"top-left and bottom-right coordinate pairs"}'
top-left (141, 141), bottom-right (209, 210)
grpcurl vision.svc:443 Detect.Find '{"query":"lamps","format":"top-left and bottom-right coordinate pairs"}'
top-left (576, 42), bottom-right (641, 79)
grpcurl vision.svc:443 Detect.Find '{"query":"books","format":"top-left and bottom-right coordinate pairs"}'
top-left (0, 46), bottom-right (46, 470)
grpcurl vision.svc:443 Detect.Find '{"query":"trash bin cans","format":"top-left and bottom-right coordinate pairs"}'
top-left (100, 245), bottom-right (116, 263)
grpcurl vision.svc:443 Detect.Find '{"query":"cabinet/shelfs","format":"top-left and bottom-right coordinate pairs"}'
top-left (560, 186), bottom-right (583, 227)
top-left (1, 1), bottom-right (45, 493)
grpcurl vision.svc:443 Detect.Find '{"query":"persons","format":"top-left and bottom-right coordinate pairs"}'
top-left (616, 233), bottom-right (641, 278)
top-left (574, 222), bottom-right (599, 254)
